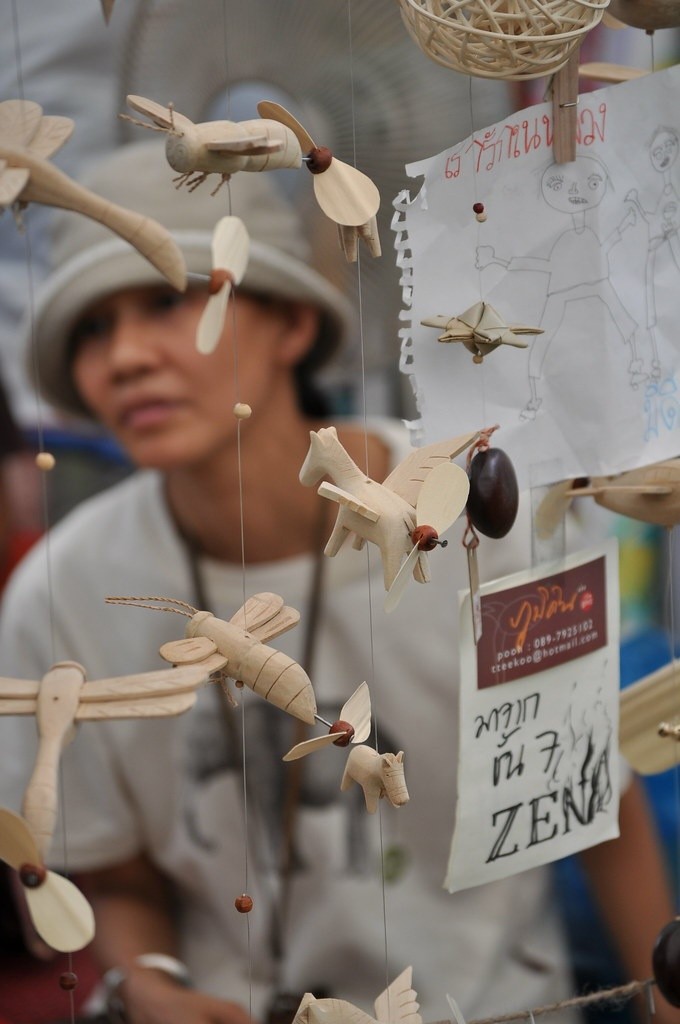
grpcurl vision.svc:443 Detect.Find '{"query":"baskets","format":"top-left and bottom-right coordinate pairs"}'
top-left (400, 0), bottom-right (611, 80)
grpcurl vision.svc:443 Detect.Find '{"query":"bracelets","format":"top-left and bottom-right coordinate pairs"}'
top-left (104, 954), bottom-right (189, 1014)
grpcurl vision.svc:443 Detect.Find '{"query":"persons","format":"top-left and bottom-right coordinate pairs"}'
top-left (1, 144), bottom-right (680, 1024)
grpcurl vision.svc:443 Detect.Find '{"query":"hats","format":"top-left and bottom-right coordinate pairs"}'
top-left (26, 138), bottom-right (352, 420)
